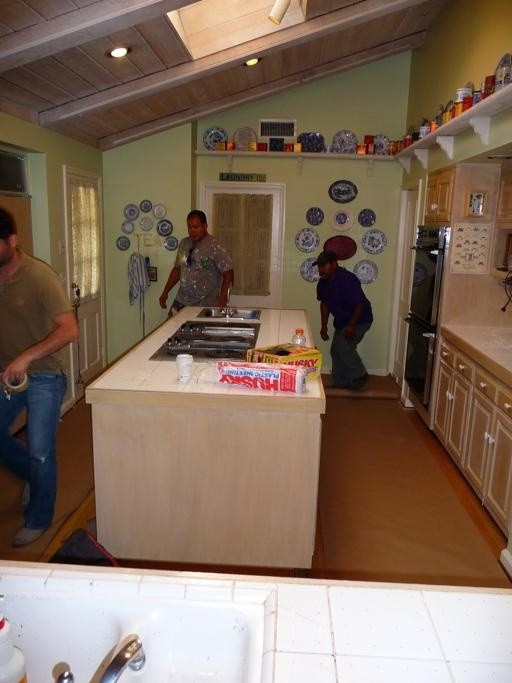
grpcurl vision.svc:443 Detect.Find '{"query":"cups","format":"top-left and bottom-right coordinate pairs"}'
top-left (176, 353), bottom-right (193, 382)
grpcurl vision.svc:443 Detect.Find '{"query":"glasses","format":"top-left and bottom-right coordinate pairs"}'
top-left (187, 248), bottom-right (193, 262)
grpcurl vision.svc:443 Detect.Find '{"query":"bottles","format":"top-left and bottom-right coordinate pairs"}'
top-left (0, 594), bottom-right (28, 683)
top-left (291, 328), bottom-right (306, 347)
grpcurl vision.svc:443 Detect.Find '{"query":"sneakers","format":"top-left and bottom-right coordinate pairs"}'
top-left (22, 480), bottom-right (32, 505)
top-left (352, 374), bottom-right (369, 389)
top-left (12, 526), bottom-right (43, 546)
top-left (332, 383), bottom-right (350, 388)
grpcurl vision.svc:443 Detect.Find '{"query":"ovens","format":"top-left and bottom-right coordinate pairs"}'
top-left (403, 224), bottom-right (447, 410)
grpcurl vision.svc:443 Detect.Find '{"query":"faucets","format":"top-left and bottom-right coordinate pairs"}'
top-left (225, 286), bottom-right (234, 317)
top-left (52, 633), bottom-right (147, 683)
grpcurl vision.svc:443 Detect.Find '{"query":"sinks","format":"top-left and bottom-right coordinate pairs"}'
top-left (196, 307), bottom-right (261, 319)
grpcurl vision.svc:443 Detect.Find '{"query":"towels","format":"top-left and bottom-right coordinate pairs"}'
top-left (129, 254), bottom-right (148, 305)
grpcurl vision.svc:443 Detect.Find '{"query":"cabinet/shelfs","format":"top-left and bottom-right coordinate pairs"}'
top-left (423, 163), bottom-right (501, 222)
top-left (431, 326), bottom-right (511, 571)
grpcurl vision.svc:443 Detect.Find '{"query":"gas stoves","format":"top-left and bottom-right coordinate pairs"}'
top-left (148, 320), bottom-right (261, 362)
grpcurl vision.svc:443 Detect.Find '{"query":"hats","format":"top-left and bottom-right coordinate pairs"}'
top-left (312, 249), bottom-right (337, 264)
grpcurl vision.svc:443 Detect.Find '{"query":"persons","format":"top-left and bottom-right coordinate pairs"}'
top-left (0, 209), bottom-right (79, 545)
top-left (159, 210), bottom-right (233, 317)
top-left (313, 251), bottom-right (373, 387)
